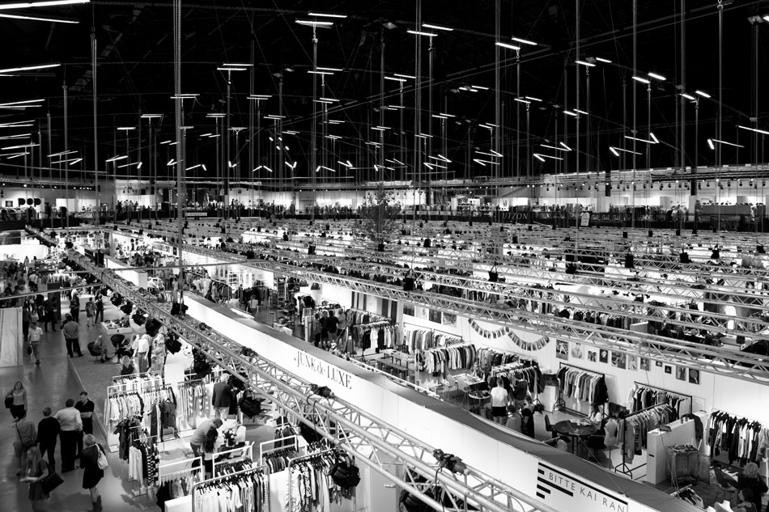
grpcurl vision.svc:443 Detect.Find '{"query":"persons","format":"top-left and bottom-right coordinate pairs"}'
top-left (1, 200), bottom-right (769, 512)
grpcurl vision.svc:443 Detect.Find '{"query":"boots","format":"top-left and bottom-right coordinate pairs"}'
top-left (87, 495), bottom-right (102, 512)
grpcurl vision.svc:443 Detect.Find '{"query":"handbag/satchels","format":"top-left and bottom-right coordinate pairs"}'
top-left (96, 447), bottom-right (109, 470)
top-left (26, 346), bottom-right (32, 355)
top-left (38, 472), bottom-right (64, 494)
top-left (4, 393), bottom-right (14, 409)
top-left (23, 440), bottom-right (38, 452)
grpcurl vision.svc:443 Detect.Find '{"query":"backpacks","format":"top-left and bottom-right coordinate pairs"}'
top-left (96, 298), bottom-right (104, 311)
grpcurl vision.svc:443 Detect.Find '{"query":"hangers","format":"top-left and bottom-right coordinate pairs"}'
top-left (46, 272), bottom-right (763, 500)
